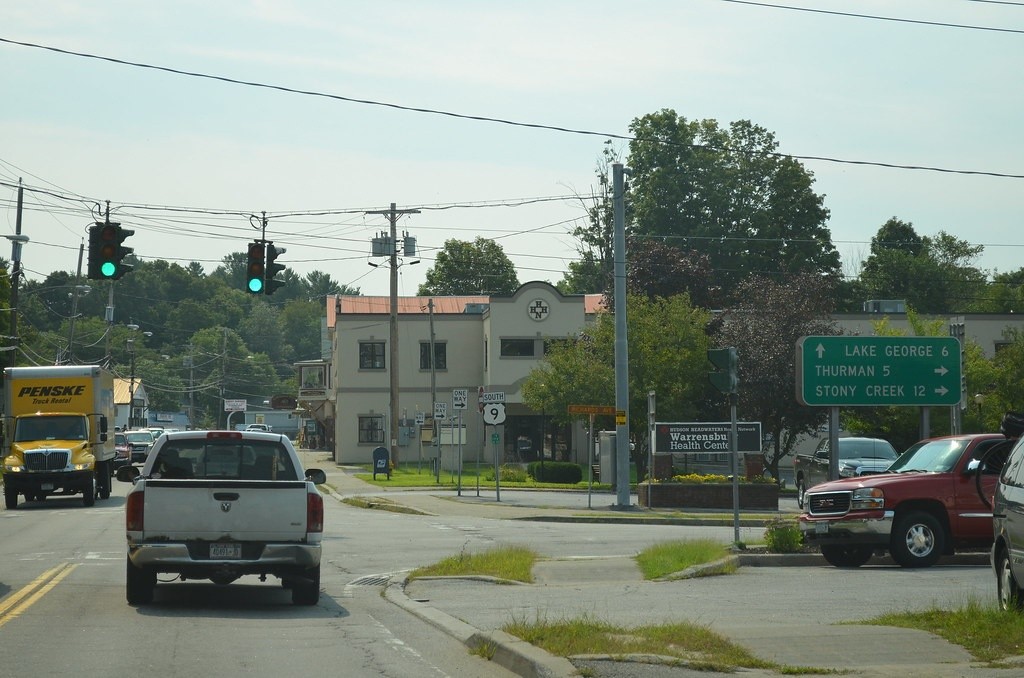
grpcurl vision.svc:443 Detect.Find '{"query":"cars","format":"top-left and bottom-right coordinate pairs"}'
top-left (195, 424), bottom-right (272, 476)
top-left (112, 428), bottom-right (179, 470)
top-left (800, 433), bottom-right (1024, 612)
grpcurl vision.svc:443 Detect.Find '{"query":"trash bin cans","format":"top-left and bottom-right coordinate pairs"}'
top-left (372, 446), bottom-right (390, 481)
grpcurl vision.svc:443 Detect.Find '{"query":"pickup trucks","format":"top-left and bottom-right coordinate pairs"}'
top-left (794, 437), bottom-right (899, 510)
top-left (116, 430), bottom-right (326, 608)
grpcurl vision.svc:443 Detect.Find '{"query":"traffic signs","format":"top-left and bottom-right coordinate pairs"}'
top-left (415, 390), bottom-right (467, 425)
top-left (803, 335), bottom-right (961, 406)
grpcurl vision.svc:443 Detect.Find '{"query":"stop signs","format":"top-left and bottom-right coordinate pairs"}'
top-left (478, 386), bottom-right (484, 413)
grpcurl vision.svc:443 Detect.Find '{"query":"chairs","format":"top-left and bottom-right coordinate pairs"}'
top-left (255, 455), bottom-right (278, 474)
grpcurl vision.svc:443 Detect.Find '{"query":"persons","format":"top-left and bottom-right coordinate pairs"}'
top-left (122, 424), bottom-right (127, 432)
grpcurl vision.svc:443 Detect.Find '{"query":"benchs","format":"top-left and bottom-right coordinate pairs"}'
top-left (592, 464), bottom-right (601, 483)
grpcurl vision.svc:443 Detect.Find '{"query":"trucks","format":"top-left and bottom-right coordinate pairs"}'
top-left (0, 364), bottom-right (116, 510)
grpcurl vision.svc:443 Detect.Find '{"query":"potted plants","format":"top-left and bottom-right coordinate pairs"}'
top-left (637, 472), bottom-right (779, 512)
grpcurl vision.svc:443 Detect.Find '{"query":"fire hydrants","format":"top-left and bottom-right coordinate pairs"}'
top-left (389, 459), bottom-right (394, 476)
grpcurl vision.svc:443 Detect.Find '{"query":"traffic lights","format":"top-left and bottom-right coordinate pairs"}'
top-left (118, 228), bottom-right (135, 278)
top-left (264, 245), bottom-right (287, 294)
top-left (248, 243), bottom-right (265, 294)
top-left (90, 226), bottom-right (118, 279)
top-left (707, 348), bottom-right (737, 394)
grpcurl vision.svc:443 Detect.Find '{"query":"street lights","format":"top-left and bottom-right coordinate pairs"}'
top-left (8, 285), bottom-right (92, 367)
top-left (63, 325), bottom-right (139, 366)
top-left (105, 332), bottom-right (153, 370)
top-left (975, 394), bottom-right (985, 434)
top-left (129, 354), bottom-right (170, 430)
